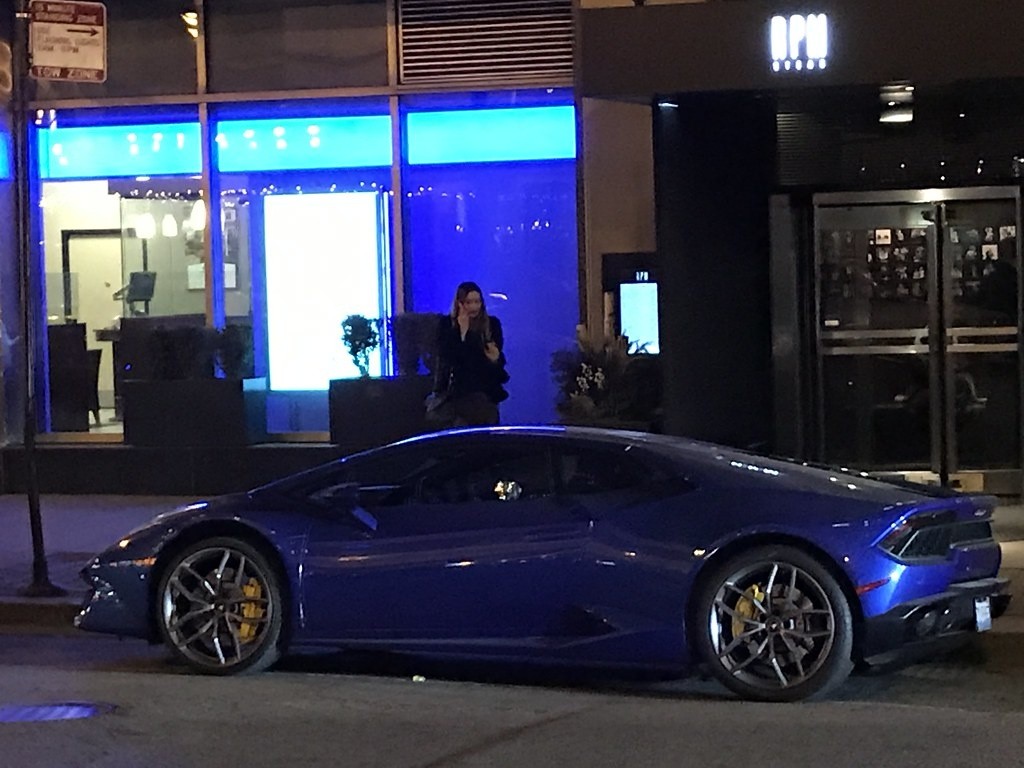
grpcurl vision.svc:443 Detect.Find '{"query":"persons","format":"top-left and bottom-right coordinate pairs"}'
top-left (837, 272), bottom-right (888, 331)
top-left (426, 282), bottom-right (509, 431)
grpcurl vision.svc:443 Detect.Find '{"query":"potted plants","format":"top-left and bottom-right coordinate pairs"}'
top-left (329, 312), bottom-right (437, 442)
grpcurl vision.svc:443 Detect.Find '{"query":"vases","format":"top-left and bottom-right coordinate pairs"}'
top-left (566, 413), bottom-right (668, 438)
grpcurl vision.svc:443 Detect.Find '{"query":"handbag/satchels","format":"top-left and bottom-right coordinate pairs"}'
top-left (425, 394), bottom-right (456, 423)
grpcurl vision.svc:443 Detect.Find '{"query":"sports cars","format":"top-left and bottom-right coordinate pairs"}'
top-left (68, 425), bottom-right (1017, 692)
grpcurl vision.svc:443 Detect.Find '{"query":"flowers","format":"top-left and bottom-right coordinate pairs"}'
top-left (550, 320), bottom-right (666, 421)
